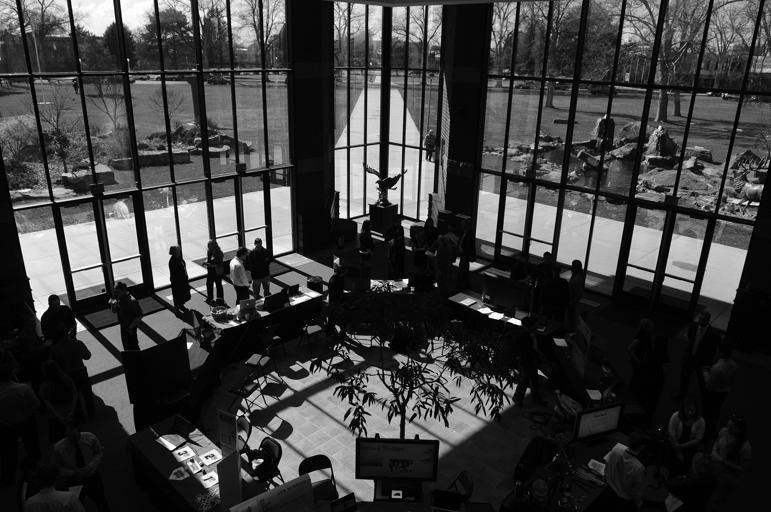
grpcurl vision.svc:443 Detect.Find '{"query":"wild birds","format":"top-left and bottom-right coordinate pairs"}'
top-left (363, 163), bottom-right (409, 190)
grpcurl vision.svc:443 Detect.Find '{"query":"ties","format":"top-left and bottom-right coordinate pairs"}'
top-left (75, 446), bottom-right (85, 468)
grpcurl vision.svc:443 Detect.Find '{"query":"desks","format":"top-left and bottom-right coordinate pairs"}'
top-left (129, 411), bottom-right (269, 512)
top-left (200, 283), bottom-right (324, 365)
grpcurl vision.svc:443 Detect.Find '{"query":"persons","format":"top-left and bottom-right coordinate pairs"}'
top-left (425, 129), bottom-right (435, 162)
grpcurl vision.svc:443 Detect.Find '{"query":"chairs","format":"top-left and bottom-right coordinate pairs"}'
top-left (448, 469), bottom-right (474, 501)
top-left (298, 454), bottom-right (338, 511)
top-left (249, 437), bottom-right (284, 491)
top-left (264, 322), bottom-right (289, 361)
top-left (237, 415), bottom-right (252, 460)
top-left (226, 370), bottom-right (269, 415)
top-left (120, 350), bottom-right (173, 413)
top-left (244, 342), bottom-right (277, 386)
top-left (298, 311), bottom-right (325, 345)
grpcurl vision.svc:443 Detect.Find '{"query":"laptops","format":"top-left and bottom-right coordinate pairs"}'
top-left (256, 291), bottom-right (284, 310)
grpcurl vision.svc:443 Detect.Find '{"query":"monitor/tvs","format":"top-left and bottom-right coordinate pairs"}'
top-left (576, 402), bottom-right (622, 446)
top-left (356, 437), bottom-right (439, 481)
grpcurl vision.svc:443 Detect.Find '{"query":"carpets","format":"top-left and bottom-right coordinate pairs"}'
top-left (59, 278), bottom-right (136, 308)
top-left (193, 248), bottom-right (253, 269)
